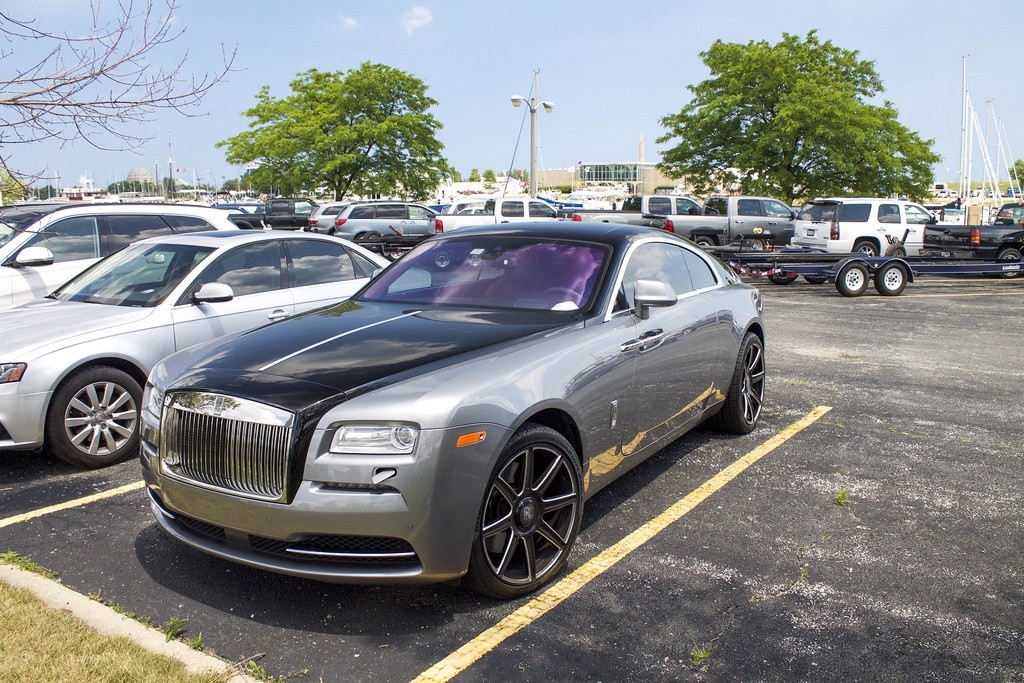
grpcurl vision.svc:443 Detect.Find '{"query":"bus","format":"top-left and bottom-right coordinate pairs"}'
top-left (934, 184), bottom-right (945, 196)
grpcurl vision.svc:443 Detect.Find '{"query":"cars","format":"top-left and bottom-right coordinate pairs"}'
top-left (0, 230), bottom-right (432, 467)
top-left (140, 220), bottom-right (767, 599)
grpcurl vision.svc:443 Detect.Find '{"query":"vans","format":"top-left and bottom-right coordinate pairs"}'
top-left (950, 191), bottom-right (958, 198)
top-left (551, 190), bottom-right (561, 196)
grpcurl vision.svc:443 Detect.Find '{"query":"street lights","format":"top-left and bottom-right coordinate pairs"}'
top-left (510, 95), bottom-right (555, 196)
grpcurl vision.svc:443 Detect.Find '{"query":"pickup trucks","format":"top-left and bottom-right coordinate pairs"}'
top-left (229, 199), bottom-right (319, 231)
top-left (641, 195), bottom-right (799, 251)
top-left (571, 195), bottom-right (704, 226)
top-left (921, 203), bottom-right (1024, 278)
top-left (428, 197), bottom-right (562, 232)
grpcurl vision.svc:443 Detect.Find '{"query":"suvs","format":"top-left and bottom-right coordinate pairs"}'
top-left (0, 202), bottom-right (244, 305)
top-left (786, 197), bottom-right (948, 258)
top-left (308, 202), bottom-right (353, 236)
top-left (332, 201), bottom-right (440, 249)
top-left (939, 190), bottom-right (947, 198)
